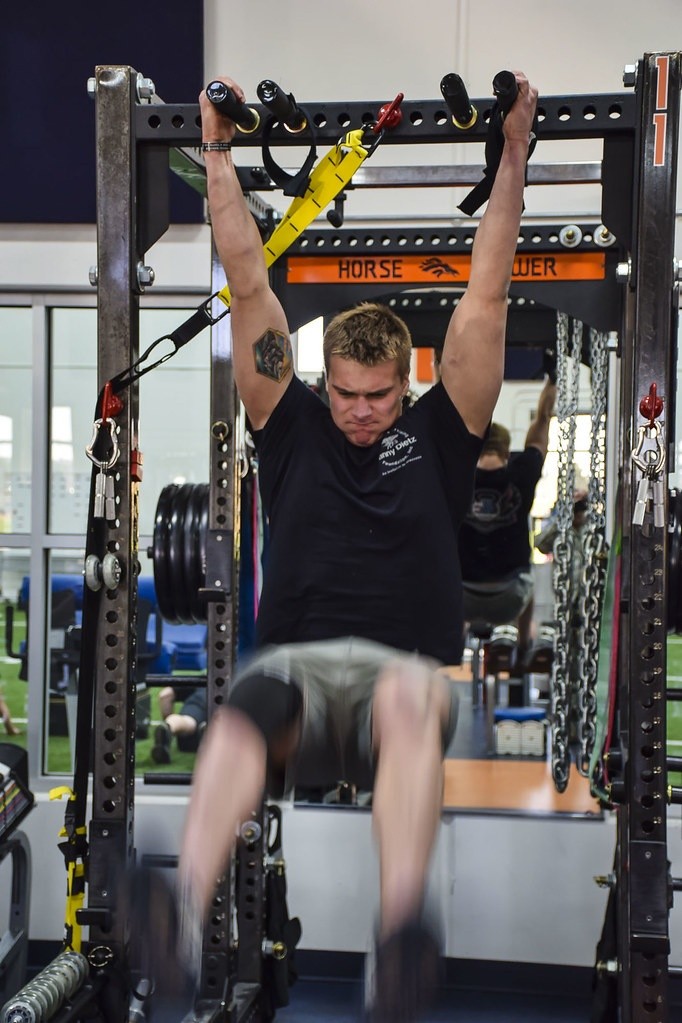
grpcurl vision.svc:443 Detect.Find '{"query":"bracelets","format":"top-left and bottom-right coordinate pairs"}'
top-left (202, 142), bottom-right (231, 152)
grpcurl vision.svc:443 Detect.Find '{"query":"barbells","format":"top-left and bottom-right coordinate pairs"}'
top-left (143, 477), bottom-right (213, 628)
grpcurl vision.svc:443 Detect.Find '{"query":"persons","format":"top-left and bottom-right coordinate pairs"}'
top-left (457, 347), bottom-right (558, 673)
top-left (0, 697), bottom-right (21, 735)
top-left (116, 71), bottom-right (538, 1023)
top-left (151, 684), bottom-right (207, 765)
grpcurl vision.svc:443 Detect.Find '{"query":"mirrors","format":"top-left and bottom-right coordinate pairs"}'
top-left (220, 253), bottom-right (619, 822)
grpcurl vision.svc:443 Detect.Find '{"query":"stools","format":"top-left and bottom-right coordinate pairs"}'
top-left (490, 705), bottom-right (552, 765)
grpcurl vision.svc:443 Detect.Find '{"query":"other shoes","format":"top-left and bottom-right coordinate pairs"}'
top-left (512, 632), bottom-right (536, 670)
top-left (107, 870), bottom-right (196, 1023)
top-left (364, 922), bottom-right (445, 1023)
top-left (472, 622), bottom-right (493, 639)
